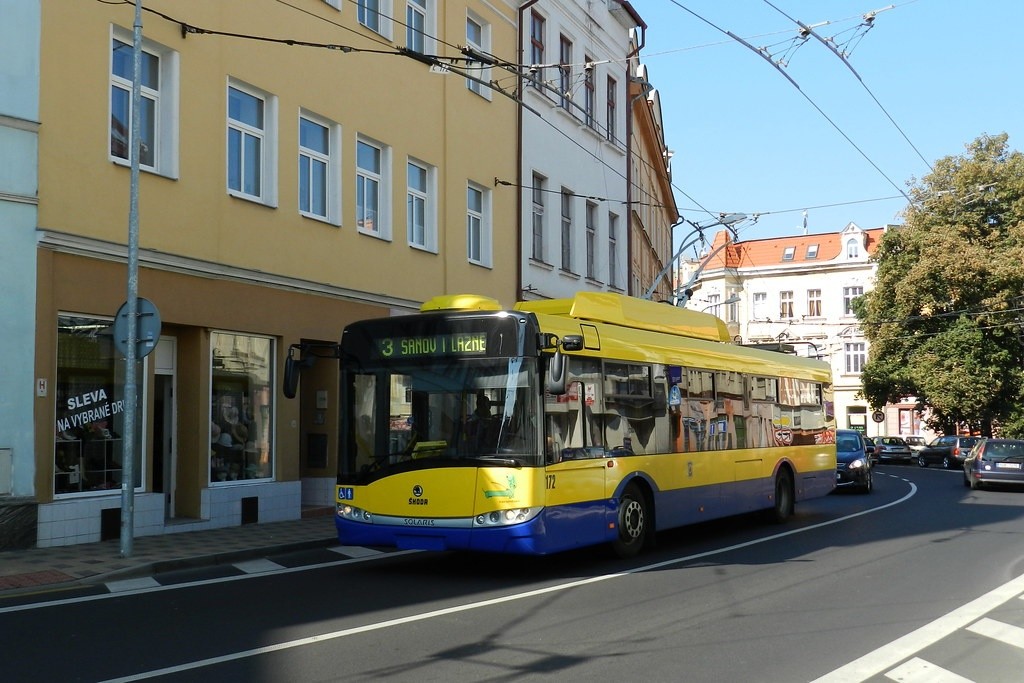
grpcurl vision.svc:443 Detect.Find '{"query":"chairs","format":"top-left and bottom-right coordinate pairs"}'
top-left (889, 440), bottom-right (895, 445)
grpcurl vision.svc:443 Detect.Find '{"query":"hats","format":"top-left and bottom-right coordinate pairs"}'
top-left (211, 400), bottom-right (259, 473)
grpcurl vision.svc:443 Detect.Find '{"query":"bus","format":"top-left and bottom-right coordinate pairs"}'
top-left (282, 290), bottom-right (837, 558)
top-left (211, 372), bottom-right (266, 475)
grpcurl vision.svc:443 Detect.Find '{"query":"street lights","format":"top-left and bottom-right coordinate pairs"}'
top-left (643, 211), bottom-right (749, 298)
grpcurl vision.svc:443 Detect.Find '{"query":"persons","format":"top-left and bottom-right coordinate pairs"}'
top-left (507, 414), bottom-right (564, 454)
top-left (228, 464), bottom-right (240, 480)
top-left (216, 466), bottom-right (228, 481)
top-left (467, 396), bottom-right (496, 448)
top-left (244, 464), bottom-right (259, 478)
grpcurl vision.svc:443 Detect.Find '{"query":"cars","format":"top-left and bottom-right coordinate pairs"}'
top-left (965, 437), bottom-right (1024, 489)
top-left (872, 436), bottom-right (913, 463)
top-left (834, 428), bottom-right (876, 493)
top-left (902, 436), bottom-right (927, 457)
top-left (863, 437), bottom-right (880, 463)
top-left (554, 445), bottom-right (637, 462)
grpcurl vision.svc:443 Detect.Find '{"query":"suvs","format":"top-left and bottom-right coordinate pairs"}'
top-left (916, 434), bottom-right (981, 468)
